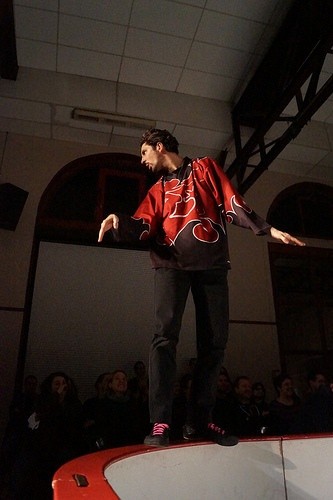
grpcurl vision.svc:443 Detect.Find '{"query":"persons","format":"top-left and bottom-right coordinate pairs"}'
top-left (9, 360), bottom-right (332, 499)
top-left (98, 129), bottom-right (305, 445)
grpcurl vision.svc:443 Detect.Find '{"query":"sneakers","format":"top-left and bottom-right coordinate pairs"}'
top-left (144, 422), bottom-right (169, 446)
top-left (183, 420), bottom-right (239, 447)
